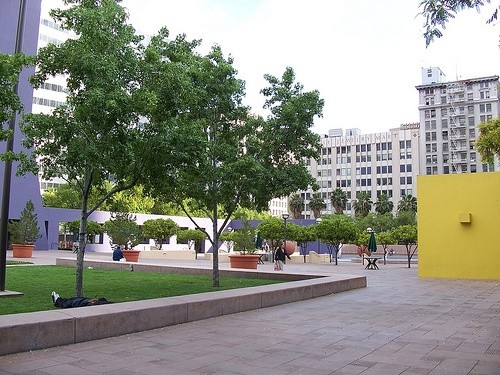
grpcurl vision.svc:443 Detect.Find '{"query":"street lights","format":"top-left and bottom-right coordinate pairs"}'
top-left (282, 212), bottom-right (290, 264)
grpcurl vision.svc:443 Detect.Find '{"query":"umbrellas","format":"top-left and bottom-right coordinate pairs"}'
top-left (366, 229), bottom-right (380, 253)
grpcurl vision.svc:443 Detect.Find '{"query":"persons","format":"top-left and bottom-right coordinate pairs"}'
top-left (271, 240), bottom-right (294, 270)
top-left (111, 243), bottom-right (130, 263)
top-left (51, 290), bottom-right (114, 308)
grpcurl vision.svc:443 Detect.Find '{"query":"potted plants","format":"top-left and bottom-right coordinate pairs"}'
top-left (9, 199), bottom-right (42, 258)
top-left (229, 215), bottom-right (259, 269)
top-left (119, 215), bottom-right (140, 262)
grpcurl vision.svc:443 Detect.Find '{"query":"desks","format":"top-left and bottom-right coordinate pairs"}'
top-left (363, 257), bottom-right (381, 270)
top-left (251, 253), bottom-right (265, 265)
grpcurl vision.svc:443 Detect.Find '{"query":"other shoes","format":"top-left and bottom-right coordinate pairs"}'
top-left (52, 291), bottom-right (60, 303)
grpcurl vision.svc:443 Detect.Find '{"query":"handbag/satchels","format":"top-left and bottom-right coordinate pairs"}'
top-left (273, 261), bottom-right (279, 272)
